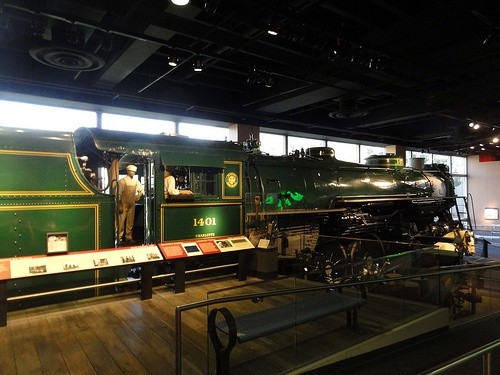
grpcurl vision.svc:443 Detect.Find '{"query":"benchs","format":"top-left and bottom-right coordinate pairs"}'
top-left (208, 277), bottom-right (369, 375)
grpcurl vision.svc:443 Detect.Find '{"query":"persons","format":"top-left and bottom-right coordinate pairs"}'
top-left (164, 168), bottom-right (194, 199)
top-left (115, 165), bottom-right (143, 245)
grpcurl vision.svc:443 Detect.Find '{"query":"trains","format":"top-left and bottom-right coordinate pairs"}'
top-left (0, 128), bottom-right (458, 327)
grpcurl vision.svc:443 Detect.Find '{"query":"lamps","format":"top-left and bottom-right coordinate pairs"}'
top-left (266, 19), bottom-right (279, 37)
top-left (333, 40), bottom-right (387, 70)
top-left (192, 54), bottom-right (204, 72)
top-left (247, 69), bottom-right (275, 89)
top-left (168, 48), bottom-right (180, 67)
